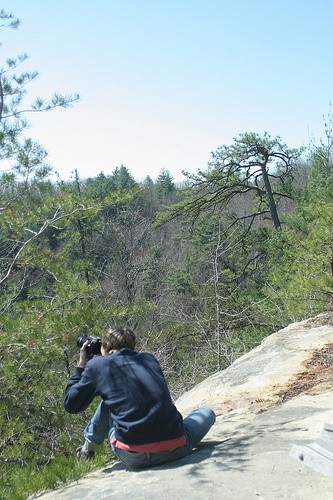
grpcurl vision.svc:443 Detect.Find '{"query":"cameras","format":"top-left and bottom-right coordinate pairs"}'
top-left (77, 336), bottom-right (102, 356)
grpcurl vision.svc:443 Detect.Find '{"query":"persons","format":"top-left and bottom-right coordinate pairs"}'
top-left (63, 325), bottom-right (215, 468)
